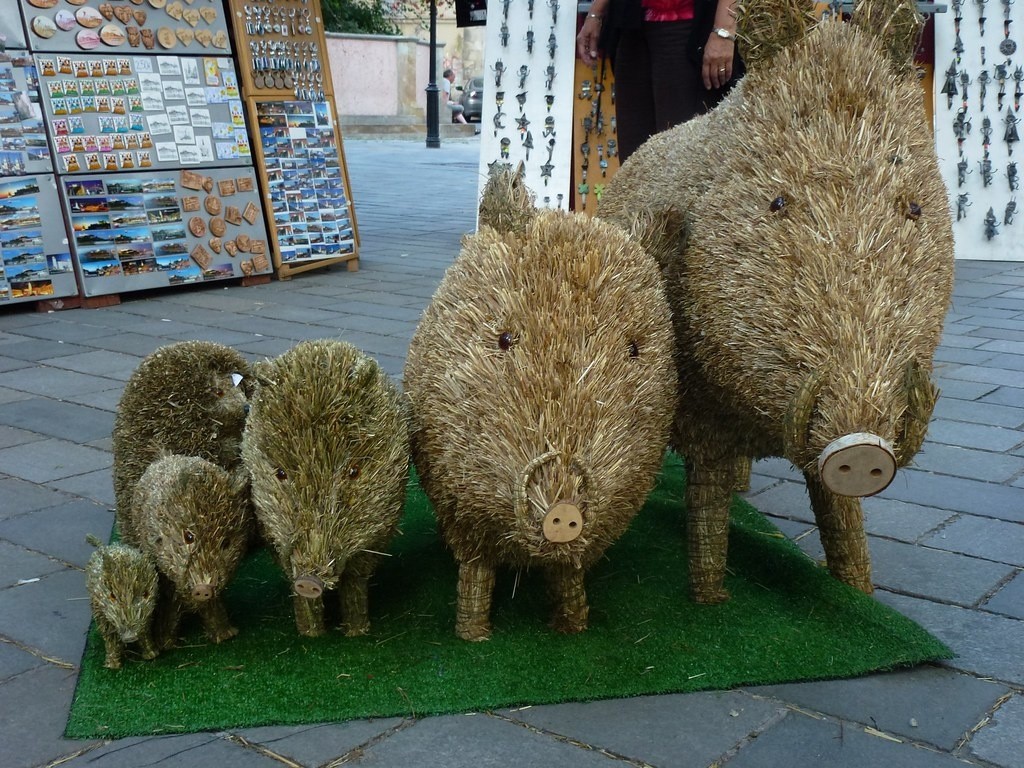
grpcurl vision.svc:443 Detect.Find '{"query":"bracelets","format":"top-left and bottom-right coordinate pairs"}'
top-left (584, 14), bottom-right (603, 23)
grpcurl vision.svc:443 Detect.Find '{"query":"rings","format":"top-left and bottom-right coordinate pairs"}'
top-left (718, 68), bottom-right (726, 72)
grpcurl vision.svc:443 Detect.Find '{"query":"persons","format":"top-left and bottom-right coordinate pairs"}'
top-left (577, 0), bottom-right (750, 171)
top-left (442, 68), bottom-right (481, 136)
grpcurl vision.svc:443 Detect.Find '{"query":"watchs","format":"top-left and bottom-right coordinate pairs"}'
top-left (710, 28), bottom-right (737, 43)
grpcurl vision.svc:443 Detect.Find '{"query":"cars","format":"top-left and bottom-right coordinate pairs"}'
top-left (456, 76), bottom-right (483, 124)
